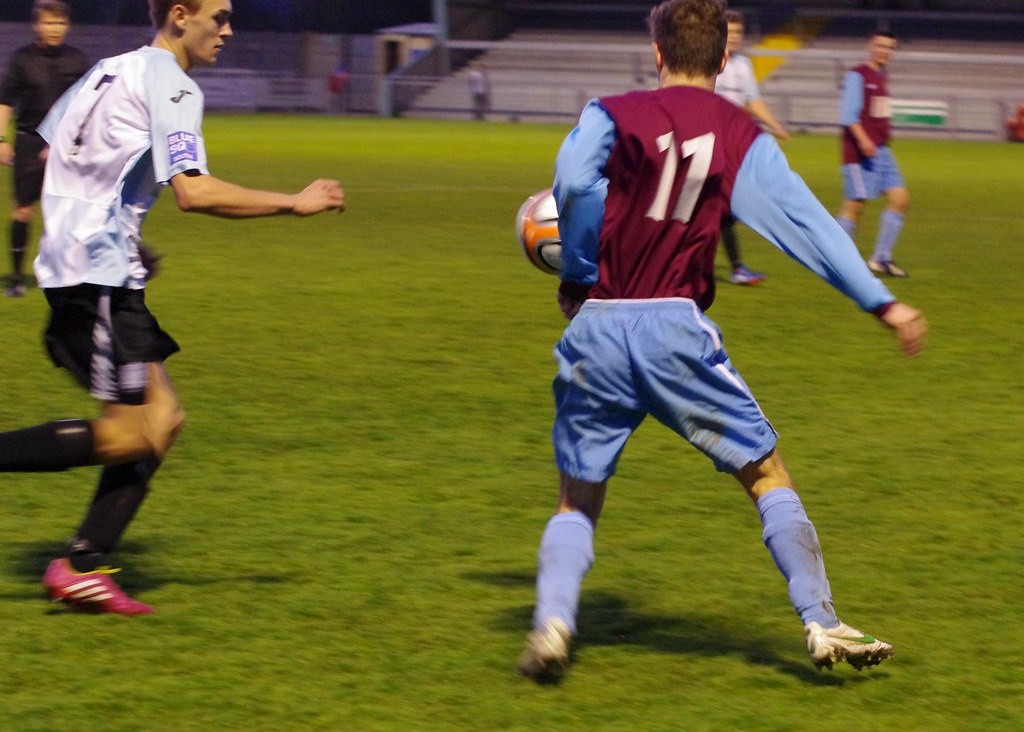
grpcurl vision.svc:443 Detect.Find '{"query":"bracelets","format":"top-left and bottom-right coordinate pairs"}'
top-left (0, 136), bottom-right (7, 143)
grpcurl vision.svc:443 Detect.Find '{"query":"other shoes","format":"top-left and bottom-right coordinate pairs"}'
top-left (9, 277), bottom-right (26, 296)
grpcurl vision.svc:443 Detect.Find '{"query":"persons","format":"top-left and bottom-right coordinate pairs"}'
top-left (836, 30), bottom-right (911, 277)
top-left (327, 67), bottom-right (349, 113)
top-left (517, 0), bottom-right (928, 684)
top-left (468, 63), bottom-right (489, 120)
top-left (0, 0), bottom-right (344, 615)
top-left (0, 0), bottom-right (92, 296)
top-left (714, 12), bottom-right (788, 284)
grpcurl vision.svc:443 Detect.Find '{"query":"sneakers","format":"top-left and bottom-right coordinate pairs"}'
top-left (516, 617), bottom-right (570, 680)
top-left (44, 559), bottom-right (153, 615)
top-left (730, 265), bottom-right (767, 284)
top-left (868, 259), bottom-right (907, 277)
top-left (805, 619), bottom-right (895, 669)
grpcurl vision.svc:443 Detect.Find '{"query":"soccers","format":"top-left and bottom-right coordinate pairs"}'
top-left (518, 185), bottom-right (565, 274)
